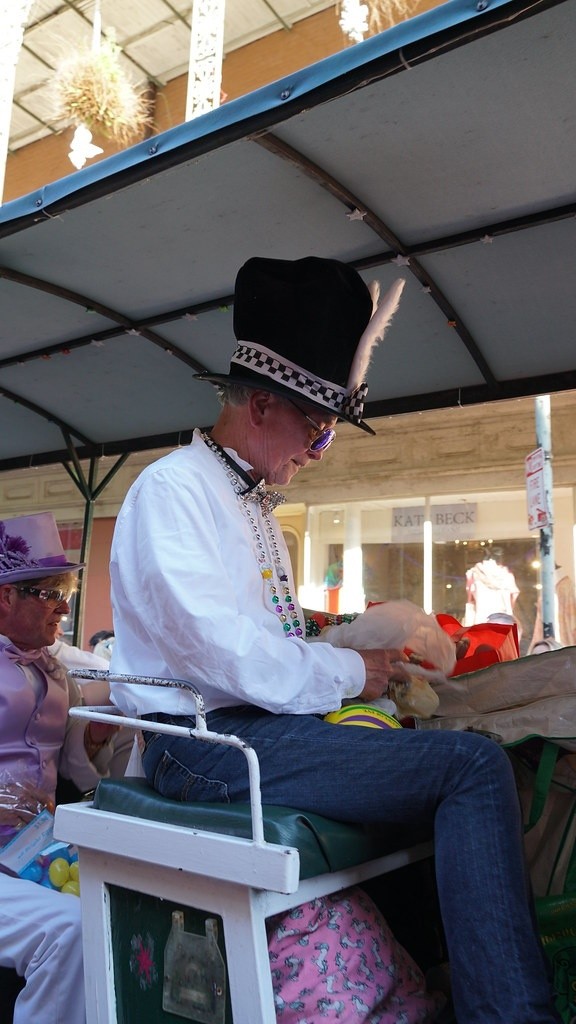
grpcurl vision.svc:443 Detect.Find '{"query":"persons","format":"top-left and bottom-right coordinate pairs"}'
top-left (0, 555), bottom-right (104, 1023)
top-left (110, 341), bottom-right (569, 1024)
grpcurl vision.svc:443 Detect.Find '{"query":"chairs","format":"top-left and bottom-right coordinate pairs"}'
top-left (48, 664), bottom-right (438, 1024)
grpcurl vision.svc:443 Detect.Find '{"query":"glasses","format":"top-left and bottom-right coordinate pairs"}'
top-left (15, 583), bottom-right (73, 610)
top-left (270, 390), bottom-right (336, 453)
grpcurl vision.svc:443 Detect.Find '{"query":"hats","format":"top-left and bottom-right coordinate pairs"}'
top-left (0, 511), bottom-right (88, 587)
top-left (191, 255), bottom-right (379, 438)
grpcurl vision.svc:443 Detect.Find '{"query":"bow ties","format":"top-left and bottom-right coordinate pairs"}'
top-left (2, 640), bottom-right (44, 668)
top-left (235, 477), bottom-right (287, 517)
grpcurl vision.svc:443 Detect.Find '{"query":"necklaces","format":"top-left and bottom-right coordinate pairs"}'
top-left (202, 430), bottom-right (305, 640)
